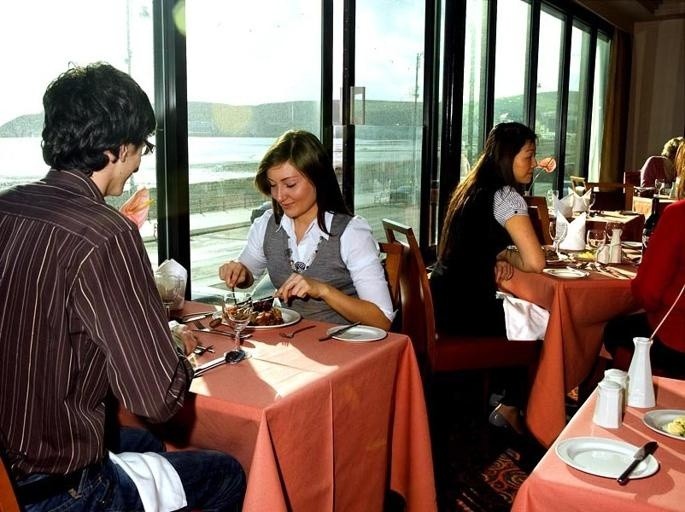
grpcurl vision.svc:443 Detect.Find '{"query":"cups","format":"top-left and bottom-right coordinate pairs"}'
top-left (164, 298), bottom-right (176, 321)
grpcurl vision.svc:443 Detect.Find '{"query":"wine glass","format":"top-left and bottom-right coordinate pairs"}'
top-left (544, 186), bottom-right (624, 264)
top-left (223, 293), bottom-right (254, 360)
top-left (654, 176), bottom-right (665, 196)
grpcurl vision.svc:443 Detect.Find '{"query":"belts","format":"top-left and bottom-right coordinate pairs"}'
top-left (17, 461), bottom-right (103, 508)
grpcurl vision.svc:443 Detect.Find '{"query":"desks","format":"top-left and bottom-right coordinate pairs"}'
top-left (494, 245), bottom-right (642, 446)
top-left (510, 368), bottom-right (685, 512)
top-left (118, 294), bottom-right (439, 510)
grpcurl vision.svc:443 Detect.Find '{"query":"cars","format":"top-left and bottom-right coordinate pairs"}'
top-left (249, 201), bottom-right (273, 225)
top-left (564, 163), bottom-right (574, 179)
top-left (389, 185), bottom-right (420, 200)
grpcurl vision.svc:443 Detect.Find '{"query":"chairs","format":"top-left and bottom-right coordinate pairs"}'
top-left (386, 219), bottom-right (544, 407)
top-left (371, 235), bottom-right (408, 301)
top-left (499, 151), bottom-right (684, 242)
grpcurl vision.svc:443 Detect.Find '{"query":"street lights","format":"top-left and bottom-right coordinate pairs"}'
top-left (122, 0), bottom-right (149, 198)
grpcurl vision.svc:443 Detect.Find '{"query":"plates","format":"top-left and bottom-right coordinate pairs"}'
top-left (622, 241), bottom-right (644, 248)
top-left (326, 324), bottom-right (388, 342)
top-left (640, 409), bottom-right (685, 442)
top-left (542, 268), bottom-right (587, 280)
top-left (211, 304), bottom-right (301, 332)
top-left (554, 436), bottom-right (660, 481)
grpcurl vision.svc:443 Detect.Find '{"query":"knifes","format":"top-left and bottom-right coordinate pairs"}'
top-left (318, 320), bottom-right (362, 342)
top-left (616, 440), bottom-right (658, 484)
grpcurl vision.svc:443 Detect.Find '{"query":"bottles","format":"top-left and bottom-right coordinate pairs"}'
top-left (668, 181), bottom-right (678, 199)
top-left (642, 196), bottom-right (659, 255)
top-left (592, 334), bottom-right (657, 428)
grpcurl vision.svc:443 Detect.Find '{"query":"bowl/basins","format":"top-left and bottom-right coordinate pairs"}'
top-left (634, 186), bottom-right (655, 198)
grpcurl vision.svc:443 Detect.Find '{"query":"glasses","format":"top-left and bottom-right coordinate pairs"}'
top-left (141, 138), bottom-right (155, 157)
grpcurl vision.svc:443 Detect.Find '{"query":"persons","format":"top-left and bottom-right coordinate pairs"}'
top-left (577, 136), bottom-right (685, 411)
top-left (0, 63), bottom-right (246, 511)
top-left (219, 131), bottom-right (397, 332)
top-left (429, 122), bottom-right (547, 437)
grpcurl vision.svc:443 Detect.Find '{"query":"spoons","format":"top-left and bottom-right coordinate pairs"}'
top-left (193, 350), bottom-right (244, 377)
top-left (191, 328), bottom-right (253, 340)
top-left (278, 325), bottom-right (318, 338)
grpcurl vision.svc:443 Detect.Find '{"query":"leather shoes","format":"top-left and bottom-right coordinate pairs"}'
top-left (488, 409), bottom-right (524, 437)
